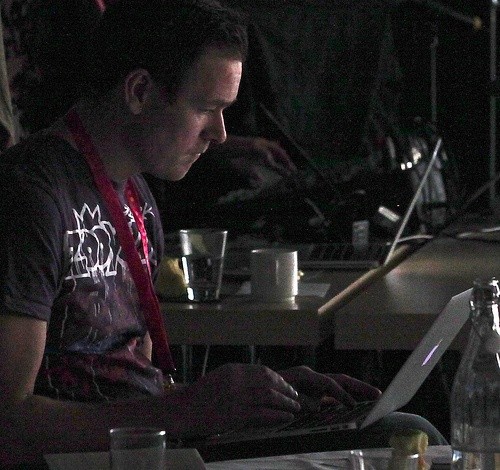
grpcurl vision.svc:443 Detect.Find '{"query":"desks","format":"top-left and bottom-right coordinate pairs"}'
top-left (333, 213), bottom-right (500, 399)
top-left (158, 228), bottom-right (406, 385)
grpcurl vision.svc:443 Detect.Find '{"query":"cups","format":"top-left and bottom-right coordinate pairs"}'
top-left (351, 448), bottom-right (420, 470)
top-left (251, 247), bottom-right (299, 304)
top-left (178, 228), bottom-right (228, 303)
top-left (109, 426), bottom-right (167, 470)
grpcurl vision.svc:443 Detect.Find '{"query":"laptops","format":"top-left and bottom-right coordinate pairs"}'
top-left (290, 138), bottom-right (443, 269)
top-left (206, 283), bottom-right (475, 446)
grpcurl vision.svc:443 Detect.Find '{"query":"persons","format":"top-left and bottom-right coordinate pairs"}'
top-left (30, 0), bottom-right (297, 236)
top-left (0, 0), bottom-right (452, 470)
top-left (330, 79), bottom-right (474, 245)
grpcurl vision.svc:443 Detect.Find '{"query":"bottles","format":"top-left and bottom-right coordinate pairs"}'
top-left (449, 280), bottom-right (500, 470)
